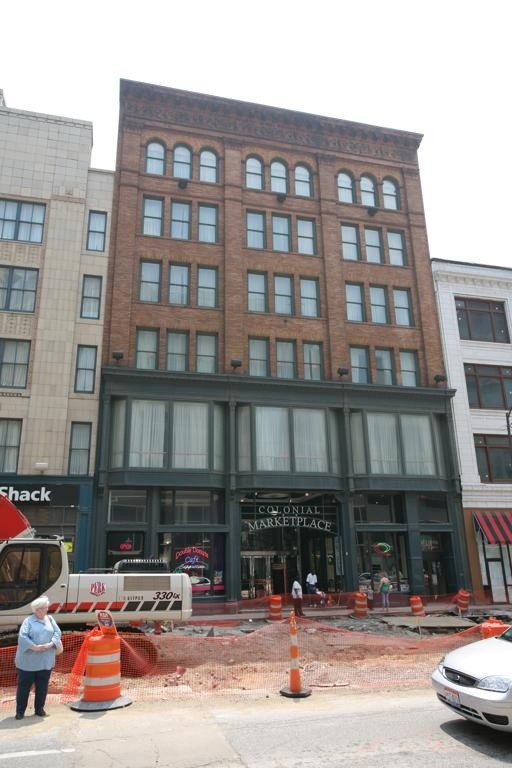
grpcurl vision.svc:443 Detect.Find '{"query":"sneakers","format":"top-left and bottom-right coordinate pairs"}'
top-left (15, 712), bottom-right (24, 719)
top-left (35, 707), bottom-right (46, 716)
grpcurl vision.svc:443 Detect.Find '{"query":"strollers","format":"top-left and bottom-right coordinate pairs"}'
top-left (309, 585), bottom-right (330, 608)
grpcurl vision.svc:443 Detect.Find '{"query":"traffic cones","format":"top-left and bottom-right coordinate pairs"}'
top-left (279, 610), bottom-right (312, 698)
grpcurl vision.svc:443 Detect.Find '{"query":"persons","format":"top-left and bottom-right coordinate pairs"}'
top-left (13, 594), bottom-right (63, 721)
top-left (377, 572), bottom-right (390, 612)
top-left (366, 583), bottom-right (375, 611)
top-left (305, 568), bottom-right (319, 608)
top-left (290, 574), bottom-right (306, 617)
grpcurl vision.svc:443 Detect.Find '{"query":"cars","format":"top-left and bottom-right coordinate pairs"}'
top-left (430, 626), bottom-right (512, 731)
top-left (192, 577), bottom-right (212, 586)
top-left (359, 573), bottom-right (383, 590)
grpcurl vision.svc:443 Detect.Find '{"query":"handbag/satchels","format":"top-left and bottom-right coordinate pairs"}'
top-left (55, 640), bottom-right (63, 657)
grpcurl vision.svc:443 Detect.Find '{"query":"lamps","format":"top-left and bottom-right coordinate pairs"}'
top-left (276, 192), bottom-right (287, 202)
top-left (34, 462), bottom-right (49, 475)
top-left (178, 178), bottom-right (188, 189)
top-left (368, 206), bottom-right (379, 216)
top-left (230, 359), bottom-right (242, 374)
top-left (112, 351), bottom-right (123, 365)
top-left (337, 367), bottom-right (349, 382)
top-left (434, 375), bottom-right (446, 387)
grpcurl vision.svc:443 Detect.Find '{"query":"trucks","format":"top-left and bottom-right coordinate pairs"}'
top-left (0, 537), bottom-right (192, 633)
